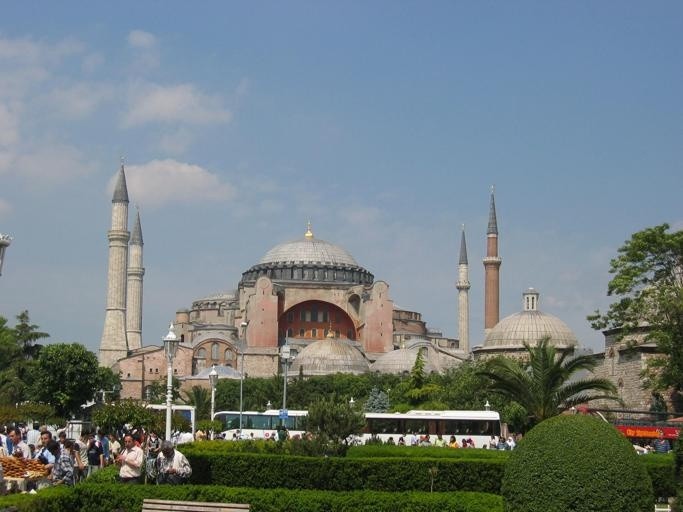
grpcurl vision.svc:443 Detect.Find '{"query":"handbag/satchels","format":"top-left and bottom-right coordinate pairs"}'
top-left (36, 454), bottom-right (51, 476)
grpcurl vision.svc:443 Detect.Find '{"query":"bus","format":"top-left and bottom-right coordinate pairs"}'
top-left (212, 406), bottom-right (327, 441)
top-left (336, 408), bottom-right (501, 451)
top-left (564, 403), bottom-right (683, 457)
top-left (111, 403), bottom-right (196, 446)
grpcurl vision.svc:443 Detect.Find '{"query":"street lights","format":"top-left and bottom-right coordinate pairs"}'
top-left (483, 400), bottom-right (491, 411)
top-left (141, 386), bottom-right (152, 404)
top-left (206, 364), bottom-right (219, 441)
top-left (162, 323), bottom-right (180, 442)
top-left (264, 398), bottom-right (272, 410)
top-left (385, 387), bottom-right (391, 404)
top-left (237, 321), bottom-right (247, 440)
top-left (346, 396), bottom-right (357, 408)
top-left (94, 390), bottom-right (104, 427)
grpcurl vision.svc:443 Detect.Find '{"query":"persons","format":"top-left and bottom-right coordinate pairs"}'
top-left (223, 434), bottom-right (226, 440)
top-left (633, 428), bottom-right (671, 458)
top-left (195, 427), bottom-right (206, 441)
top-left (435, 431), bottom-right (517, 451)
top-left (421, 435), bottom-right (432, 446)
top-left (399, 433), bottom-right (407, 446)
top-left (270, 433), bottom-right (276, 441)
top-left (411, 432), bottom-right (419, 446)
top-left (0, 420), bottom-right (192, 485)
top-left (231, 433), bottom-right (236, 441)
top-left (248, 433), bottom-right (253, 439)
top-left (388, 437), bottom-right (395, 444)
